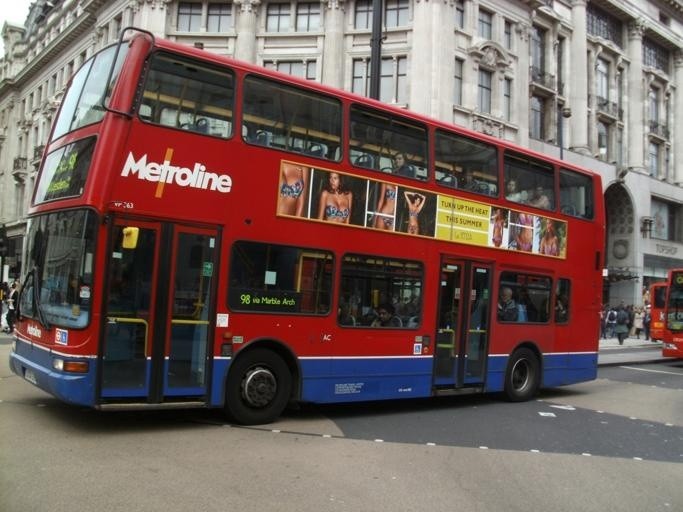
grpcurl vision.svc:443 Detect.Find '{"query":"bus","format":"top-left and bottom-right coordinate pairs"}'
top-left (648, 282), bottom-right (674, 341)
top-left (657, 266), bottom-right (682, 361)
top-left (6, 24), bottom-right (609, 429)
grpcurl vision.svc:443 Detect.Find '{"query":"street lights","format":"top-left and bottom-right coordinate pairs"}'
top-left (556, 106), bottom-right (571, 163)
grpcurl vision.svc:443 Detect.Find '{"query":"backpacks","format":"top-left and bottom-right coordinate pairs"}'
top-left (609, 311), bottom-right (616, 321)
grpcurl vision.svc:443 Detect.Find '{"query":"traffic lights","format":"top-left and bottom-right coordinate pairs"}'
top-left (0, 225), bottom-right (7, 257)
top-left (7, 239), bottom-right (16, 258)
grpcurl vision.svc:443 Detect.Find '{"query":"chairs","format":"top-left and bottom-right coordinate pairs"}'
top-left (347, 314), bottom-right (419, 328)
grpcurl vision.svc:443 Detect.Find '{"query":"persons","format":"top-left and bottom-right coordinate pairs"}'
top-left (316, 172), bottom-right (353, 225)
top-left (13, 276), bottom-right (20, 295)
top-left (459, 167), bottom-right (479, 192)
top-left (402, 190), bottom-right (426, 234)
top-left (3, 281), bottom-right (18, 335)
top-left (391, 151), bottom-right (410, 177)
top-left (372, 183), bottom-right (396, 232)
top-left (503, 178), bottom-right (522, 205)
top-left (275, 162), bottom-right (309, 218)
top-left (538, 218), bottom-right (560, 257)
top-left (495, 286), bottom-right (518, 322)
top-left (336, 293), bottom-right (419, 329)
top-left (553, 294), bottom-right (568, 322)
top-left (490, 208), bottom-right (504, 247)
top-left (510, 213), bottom-right (533, 252)
top-left (599, 299), bottom-right (659, 345)
top-left (524, 183), bottom-right (552, 211)
